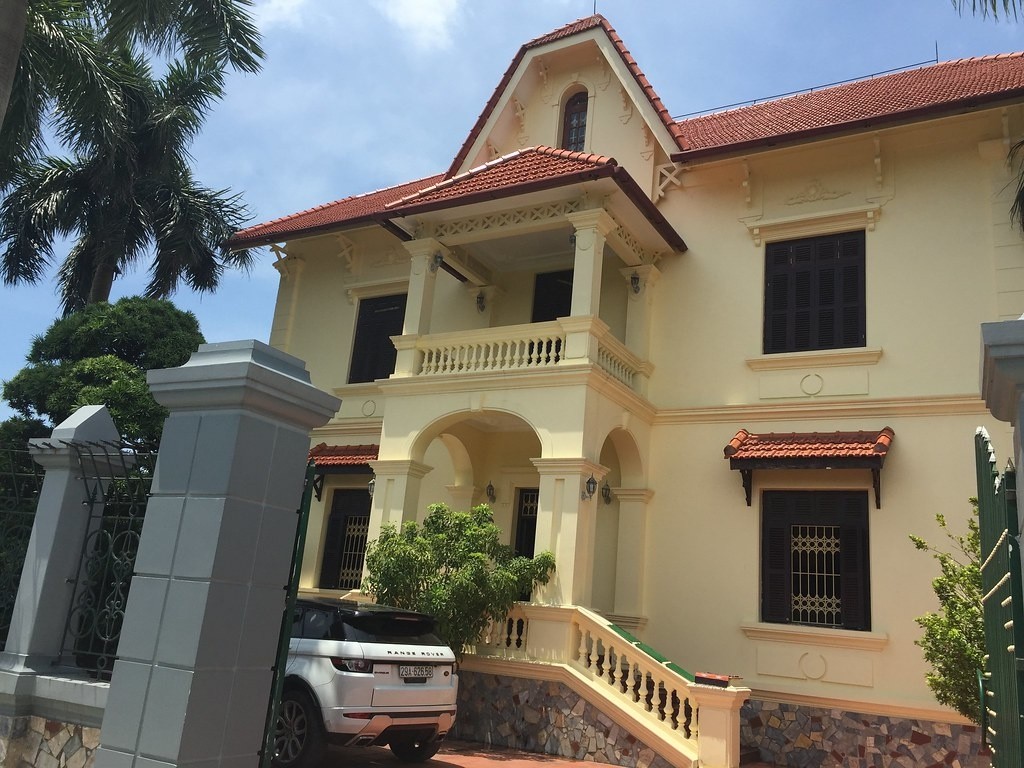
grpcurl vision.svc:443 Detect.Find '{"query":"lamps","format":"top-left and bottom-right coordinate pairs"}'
top-left (431, 250), bottom-right (443, 271)
top-left (486, 480), bottom-right (495, 503)
top-left (570, 232), bottom-right (576, 249)
top-left (475, 290), bottom-right (485, 311)
top-left (601, 480), bottom-right (610, 504)
top-left (368, 475), bottom-right (375, 498)
top-left (630, 270), bottom-right (641, 293)
top-left (582, 473), bottom-right (598, 499)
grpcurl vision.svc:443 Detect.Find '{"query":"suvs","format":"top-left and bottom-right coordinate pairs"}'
top-left (272, 596), bottom-right (459, 768)
